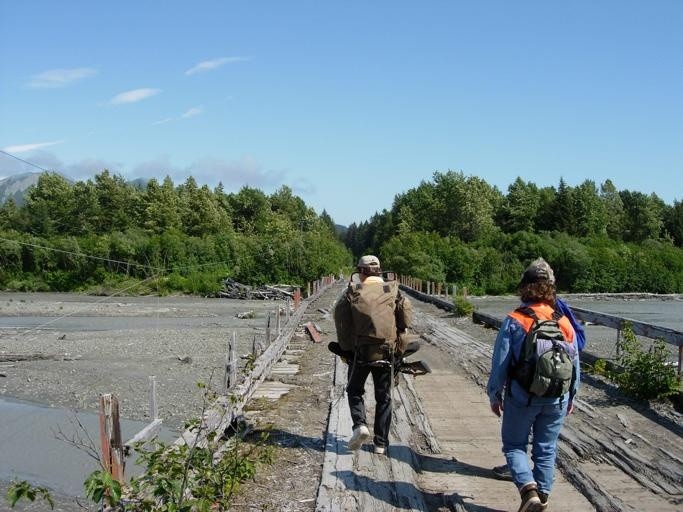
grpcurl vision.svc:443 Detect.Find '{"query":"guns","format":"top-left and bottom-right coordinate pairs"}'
top-left (396, 360), bottom-right (432, 375)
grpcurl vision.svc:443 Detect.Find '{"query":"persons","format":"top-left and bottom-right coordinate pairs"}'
top-left (486, 269), bottom-right (580, 512)
top-left (332, 253), bottom-right (413, 455)
top-left (491, 255), bottom-right (585, 482)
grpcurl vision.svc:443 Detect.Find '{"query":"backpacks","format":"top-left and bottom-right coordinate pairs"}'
top-left (344, 272), bottom-right (407, 369)
top-left (506, 308), bottom-right (576, 410)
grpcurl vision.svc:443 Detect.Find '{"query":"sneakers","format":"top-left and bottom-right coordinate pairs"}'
top-left (374, 443), bottom-right (387, 454)
top-left (492, 465), bottom-right (515, 481)
top-left (348, 425), bottom-right (370, 450)
top-left (517, 491), bottom-right (548, 512)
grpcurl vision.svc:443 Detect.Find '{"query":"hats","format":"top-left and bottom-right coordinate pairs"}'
top-left (516, 268), bottom-right (549, 289)
top-left (357, 255), bottom-right (380, 268)
